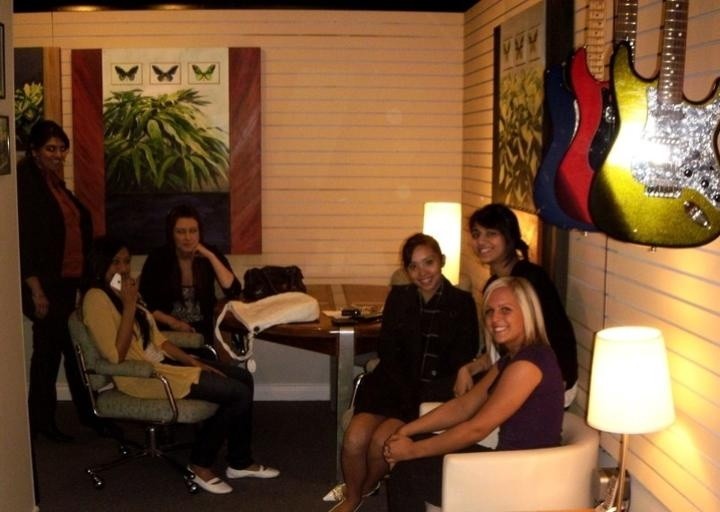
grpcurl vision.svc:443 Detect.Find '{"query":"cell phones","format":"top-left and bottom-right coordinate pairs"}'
top-left (110, 273), bottom-right (122, 291)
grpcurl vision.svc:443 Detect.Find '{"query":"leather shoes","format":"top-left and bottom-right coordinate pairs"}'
top-left (322, 482), bottom-right (381, 512)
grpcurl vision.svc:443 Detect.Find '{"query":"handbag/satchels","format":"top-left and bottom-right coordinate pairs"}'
top-left (242, 266), bottom-right (307, 301)
top-left (229, 292), bottom-right (319, 334)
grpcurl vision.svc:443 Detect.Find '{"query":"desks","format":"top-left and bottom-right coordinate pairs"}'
top-left (212, 282), bottom-right (390, 485)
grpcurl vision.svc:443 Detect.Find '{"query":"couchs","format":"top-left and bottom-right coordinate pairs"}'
top-left (413, 400), bottom-right (606, 511)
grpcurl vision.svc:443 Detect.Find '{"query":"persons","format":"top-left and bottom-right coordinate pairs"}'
top-left (16, 119), bottom-right (126, 444)
top-left (321, 232), bottom-right (480, 511)
top-left (136, 202), bottom-right (242, 360)
top-left (383, 274), bottom-right (565, 511)
top-left (452, 202), bottom-right (580, 410)
top-left (80, 232), bottom-right (281, 496)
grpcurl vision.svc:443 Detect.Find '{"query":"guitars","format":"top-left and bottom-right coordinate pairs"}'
top-left (532, 0), bottom-right (606, 231)
top-left (555, 0), bottom-right (639, 227)
top-left (586, 0), bottom-right (720, 249)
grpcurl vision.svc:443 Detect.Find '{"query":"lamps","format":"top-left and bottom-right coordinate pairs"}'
top-left (585, 323), bottom-right (678, 511)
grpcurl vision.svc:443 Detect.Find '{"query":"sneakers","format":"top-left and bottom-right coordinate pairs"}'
top-left (227, 466), bottom-right (281, 479)
top-left (184, 467), bottom-right (232, 494)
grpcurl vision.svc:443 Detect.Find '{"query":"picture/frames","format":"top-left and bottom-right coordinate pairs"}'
top-left (0, 115), bottom-right (12, 177)
top-left (0, 22), bottom-right (6, 98)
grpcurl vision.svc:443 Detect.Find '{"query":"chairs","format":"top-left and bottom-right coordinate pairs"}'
top-left (67, 303), bottom-right (222, 491)
top-left (335, 358), bottom-right (380, 478)
